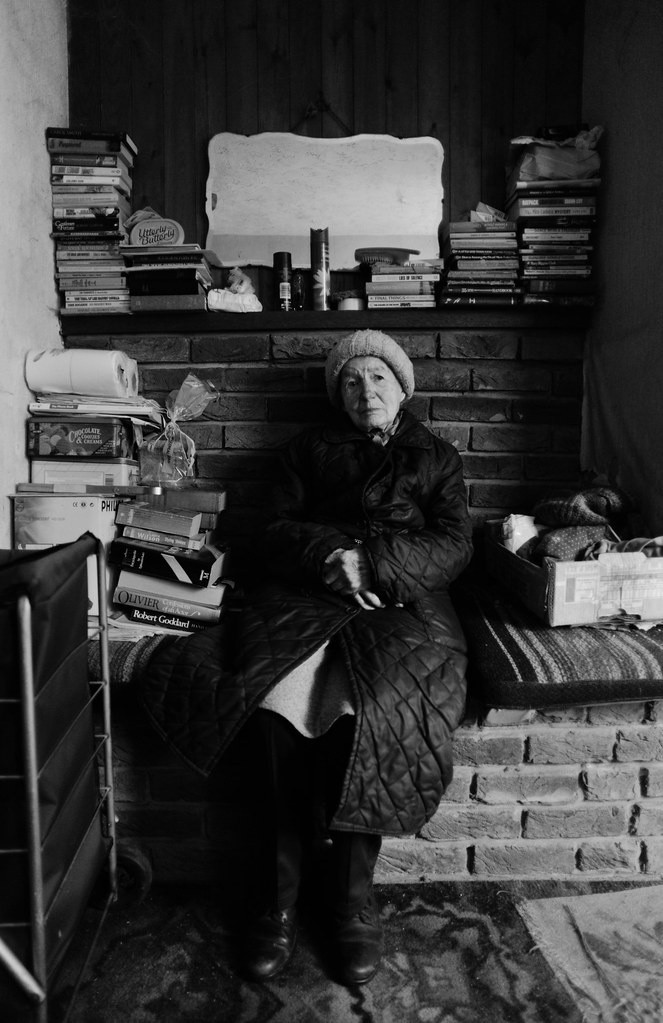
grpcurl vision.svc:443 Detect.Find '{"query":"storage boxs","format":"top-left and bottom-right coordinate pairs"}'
top-left (473, 521), bottom-right (663, 627)
top-left (8, 418), bottom-right (229, 622)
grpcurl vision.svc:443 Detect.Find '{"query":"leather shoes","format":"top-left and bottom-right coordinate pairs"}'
top-left (338, 893), bottom-right (385, 984)
top-left (239, 900), bottom-right (300, 980)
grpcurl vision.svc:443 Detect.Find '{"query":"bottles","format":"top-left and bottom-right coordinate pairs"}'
top-left (291, 269), bottom-right (306, 310)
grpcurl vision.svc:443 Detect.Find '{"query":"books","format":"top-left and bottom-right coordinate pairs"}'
top-left (444, 222), bottom-right (521, 306)
top-left (366, 265), bottom-right (442, 308)
top-left (46, 126), bottom-right (212, 316)
top-left (17, 483), bottom-right (149, 496)
top-left (28, 402), bottom-right (167, 428)
top-left (110, 487), bottom-right (230, 631)
top-left (506, 179), bottom-right (604, 310)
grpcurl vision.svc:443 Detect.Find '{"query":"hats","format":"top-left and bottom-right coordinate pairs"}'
top-left (325, 328), bottom-right (415, 409)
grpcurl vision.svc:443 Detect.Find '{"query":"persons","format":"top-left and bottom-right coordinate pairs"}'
top-left (137, 329), bottom-right (475, 982)
